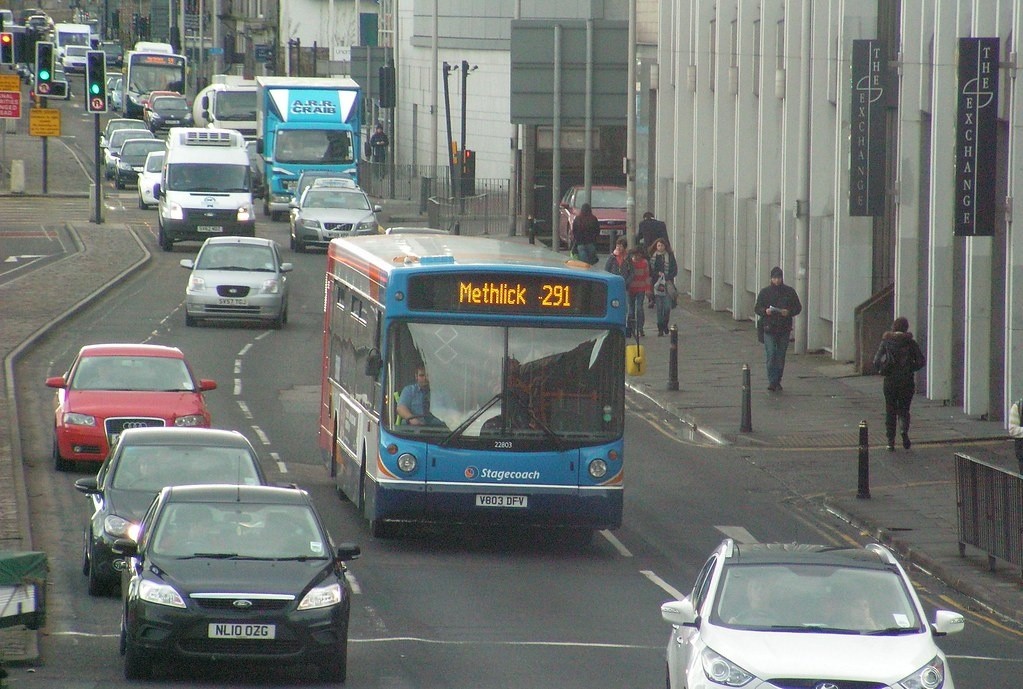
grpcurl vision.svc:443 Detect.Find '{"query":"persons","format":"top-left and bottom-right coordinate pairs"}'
top-left (873, 317), bottom-right (924, 451)
top-left (396, 361), bottom-right (458, 426)
top-left (370, 126), bottom-right (389, 180)
top-left (754, 267), bottom-right (802, 390)
top-left (648, 237), bottom-right (677, 336)
top-left (1009, 398), bottom-right (1023, 475)
top-left (604, 239), bottom-right (648, 338)
top-left (573, 203), bottom-right (599, 263)
top-left (636, 212), bottom-right (669, 307)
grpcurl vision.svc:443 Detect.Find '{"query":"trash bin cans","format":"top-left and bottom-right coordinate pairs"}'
top-left (6, 119), bottom-right (16, 133)
top-left (89, 184), bottom-right (105, 223)
top-left (10, 159), bottom-right (26, 194)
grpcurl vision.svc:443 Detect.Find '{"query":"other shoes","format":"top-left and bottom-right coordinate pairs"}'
top-left (648, 300), bottom-right (655, 309)
top-left (639, 332), bottom-right (646, 336)
top-left (658, 330), bottom-right (663, 336)
top-left (767, 383), bottom-right (782, 391)
top-left (886, 445), bottom-right (893, 451)
top-left (664, 328), bottom-right (670, 334)
top-left (901, 431), bottom-right (911, 448)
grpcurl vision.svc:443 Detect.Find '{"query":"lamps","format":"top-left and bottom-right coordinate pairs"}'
top-left (650, 63), bottom-right (785, 99)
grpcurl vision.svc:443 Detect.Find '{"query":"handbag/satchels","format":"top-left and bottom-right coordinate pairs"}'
top-left (666, 280), bottom-right (679, 301)
top-left (653, 274), bottom-right (666, 299)
top-left (877, 346), bottom-right (896, 377)
top-left (756, 320), bottom-right (766, 344)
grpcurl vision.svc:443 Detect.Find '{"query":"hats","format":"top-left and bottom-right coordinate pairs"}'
top-left (891, 317), bottom-right (909, 330)
top-left (770, 267), bottom-right (783, 278)
top-left (376, 123), bottom-right (383, 129)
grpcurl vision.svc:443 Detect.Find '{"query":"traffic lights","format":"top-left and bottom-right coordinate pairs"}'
top-left (463, 150), bottom-right (472, 175)
top-left (85, 49), bottom-right (108, 114)
top-left (0, 31), bottom-right (16, 65)
top-left (33, 40), bottom-right (68, 99)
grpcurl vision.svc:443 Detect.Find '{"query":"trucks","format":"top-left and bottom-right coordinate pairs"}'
top-left (153, 126), bottom-right (265, 252)
top-left (254, 73), bottom-right (372, 222)
top-left (192, 72), bottom-right (258, 141)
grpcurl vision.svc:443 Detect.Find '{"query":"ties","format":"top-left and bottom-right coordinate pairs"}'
top-left (421, 389), bottom-right (429, 416)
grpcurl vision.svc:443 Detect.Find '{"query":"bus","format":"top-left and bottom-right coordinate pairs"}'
top-left (121, 42), bottom-right (187, 119)
top-left (316, 232), bottom-right (647, 538)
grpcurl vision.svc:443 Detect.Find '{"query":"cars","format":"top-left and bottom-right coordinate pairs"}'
top-left (660, 537), bottom-right (967, 689)
top-left (287, 169), bottom-right (451, 253)
top-left (179, 236), bottom-right (295, 331)
top-left (97, 90), bottom-right (195, 211)
top-left (72, 425), bottom-right (297, 598)
top-left (558, 183), bottom-right (629, 251)
top-left (0, 8), bottom-right (125, 113)
top-left (112, 482), bottom-right (361, 684)
top-left (245, 139), bottom-right (265, 200)
top-left (44, 341), bottom-right (219, 472)
